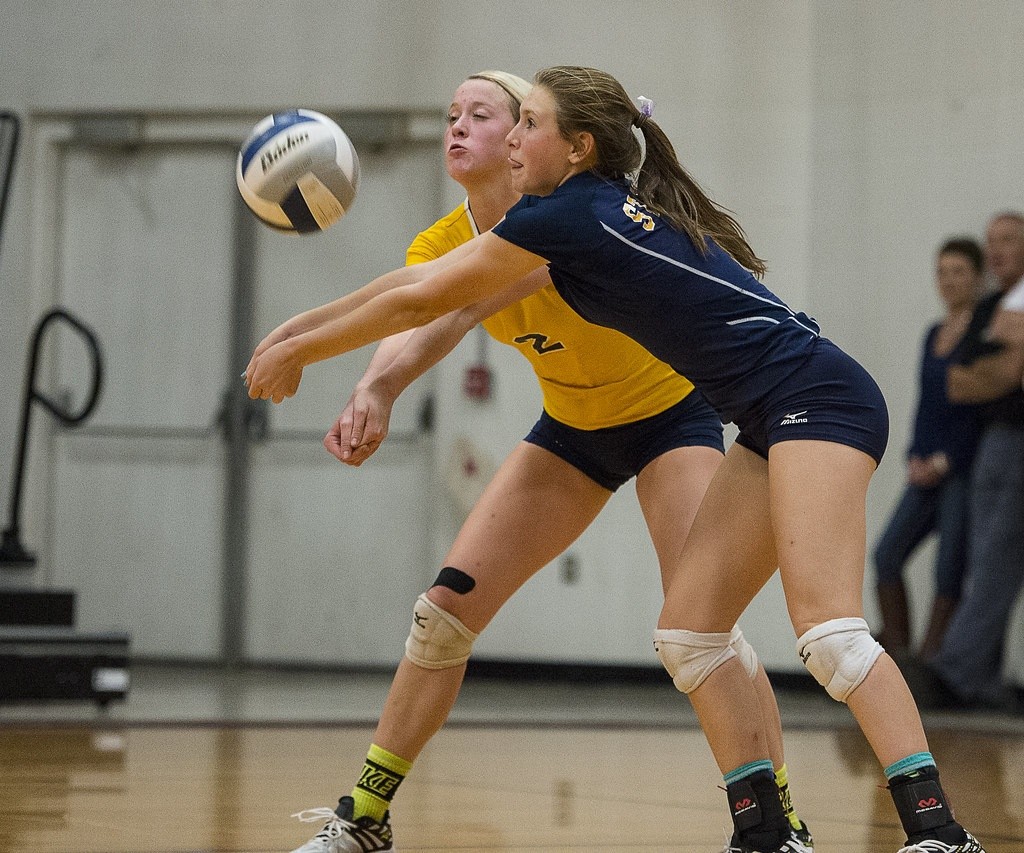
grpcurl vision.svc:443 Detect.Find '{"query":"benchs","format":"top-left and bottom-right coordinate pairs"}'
top-left (0, 530), bottom-right (135, 711)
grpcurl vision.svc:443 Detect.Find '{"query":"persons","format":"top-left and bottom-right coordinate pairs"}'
top-left (867, 213), bottom-right (1024, 705)
top-left (290, 71), bottom-right (812, 853)
top-left (241, 65), bottom-right (987, 853)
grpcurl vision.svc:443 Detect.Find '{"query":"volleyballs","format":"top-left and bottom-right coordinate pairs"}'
top-left (236, 108), bottom-right (363, 237)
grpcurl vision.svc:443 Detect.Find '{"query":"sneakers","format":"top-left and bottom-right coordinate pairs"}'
top-left (719, 828), bottom-right (811, 853)
top-left (895, 828), bottom-right (988, 853)
top-left (717, 819), bottom-right (817, 853)
top-left (288, 796), bottom-right (393, 853)
